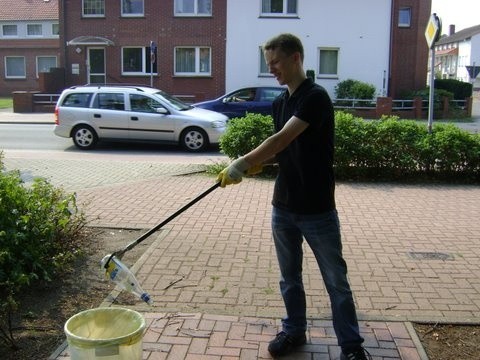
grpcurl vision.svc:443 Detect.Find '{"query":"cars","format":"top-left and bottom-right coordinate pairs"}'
top-left (54, 84), bottom-right (234, 153)
top-left (190, 85), bottom-right (288, 120)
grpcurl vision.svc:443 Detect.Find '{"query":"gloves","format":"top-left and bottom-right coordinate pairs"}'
top-left (216, 156), bottom-right (253, 188)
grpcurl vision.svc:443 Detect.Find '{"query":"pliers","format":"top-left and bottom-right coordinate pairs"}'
top-left (104, 170), bottom-right (248, 267)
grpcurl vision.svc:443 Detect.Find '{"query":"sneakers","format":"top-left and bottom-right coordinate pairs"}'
top-left (339, 346), bottom-right (373, 360)
top-left (267, 330), bottom-right (307, 360)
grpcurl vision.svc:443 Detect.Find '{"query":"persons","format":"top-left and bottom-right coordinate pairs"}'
top-left (132, 95), bottom-right (156, 112)
top-left (233, 90), bottom-right (255, 102)
top-left (216, 33), bottom-right (372, 360)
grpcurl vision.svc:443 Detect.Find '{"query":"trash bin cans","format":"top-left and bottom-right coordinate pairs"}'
top-left (64, 307), bottom-right (146, 360)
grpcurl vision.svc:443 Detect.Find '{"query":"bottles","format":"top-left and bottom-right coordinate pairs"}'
top-left (100, 253), bottom-right (152, 304)
